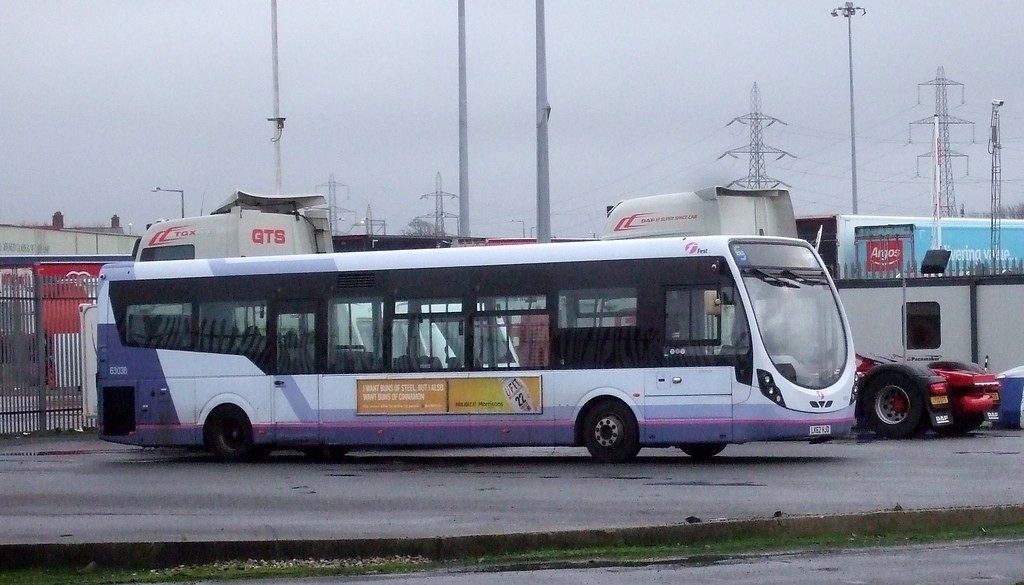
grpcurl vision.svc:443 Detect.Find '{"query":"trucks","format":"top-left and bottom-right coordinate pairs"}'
top-left (599, 182), bottom-right (1003, 438)
top-left (129, 190), bottom-right (363, 355)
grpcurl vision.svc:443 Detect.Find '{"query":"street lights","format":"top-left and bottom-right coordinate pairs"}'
top-left (830, 3), bottom-right (869, 217)
top-left (149, 186), bottom-right (186, 218)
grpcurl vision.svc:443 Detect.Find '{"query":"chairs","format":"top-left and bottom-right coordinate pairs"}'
top-left (129, 310), bottom-right (462, 372)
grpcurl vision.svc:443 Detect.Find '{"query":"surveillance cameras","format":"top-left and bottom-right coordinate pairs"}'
top-left (992, 99), bottom-right (1004, 106)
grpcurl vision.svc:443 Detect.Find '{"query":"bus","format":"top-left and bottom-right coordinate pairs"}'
top-left (93, 235), bottom-right (857, 465)
